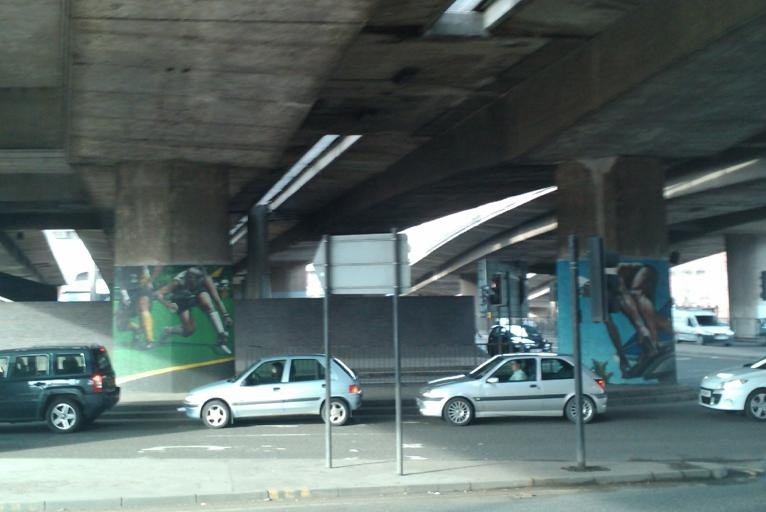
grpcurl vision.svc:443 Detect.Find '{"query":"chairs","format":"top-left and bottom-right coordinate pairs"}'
top-left (63, 360), bottom-right (76, 374)
top-left (66, 357), bottom-right (78, 367)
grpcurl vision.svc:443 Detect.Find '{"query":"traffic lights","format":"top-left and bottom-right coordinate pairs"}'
top-left (758, 270), bottom-right (766, 300)
top-left (490, 274), bottom-right (503, 304)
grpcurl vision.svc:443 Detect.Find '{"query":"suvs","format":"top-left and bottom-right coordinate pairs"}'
top-left (0, 344), bottom-right (121, 432)
top-left (487, 324), bottom-right (554, 355)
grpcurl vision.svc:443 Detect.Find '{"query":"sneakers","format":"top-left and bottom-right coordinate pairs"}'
top-left (131, 327), bottom-right (143, 344)
top-left (212, 343), bottom-right (232, 356)
top-left (159, 328), bottom-right (171, 345)
top-left (142, 341), bottom-right (154, 350)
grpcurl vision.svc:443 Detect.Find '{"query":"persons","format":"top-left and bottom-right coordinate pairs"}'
top-left (115, 278), bottom-right (232, 301)
top-left (156, 268), bottom-right (233, 356)
top-left (602, 261), bottom-right (671, 373)
top-left (269, 362), bottom-right (283, 383)
top-left (508, 360), bottom-right (528, 380)
top-left (116, 267), bottom-right (161, 351)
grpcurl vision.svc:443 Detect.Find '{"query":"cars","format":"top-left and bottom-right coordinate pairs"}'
top-left (414, 352), bottom-right (611, 425)
top-left (697, 353), bottom-right (766, 422)
top-left (183, 354), bottom-right (363, 429)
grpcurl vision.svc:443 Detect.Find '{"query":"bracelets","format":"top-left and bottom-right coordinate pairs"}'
top-left (223, 312), bottom-right (229, 317)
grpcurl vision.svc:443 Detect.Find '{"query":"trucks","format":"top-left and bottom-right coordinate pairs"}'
top-left (672, 308), bottom-right (736, 345)
